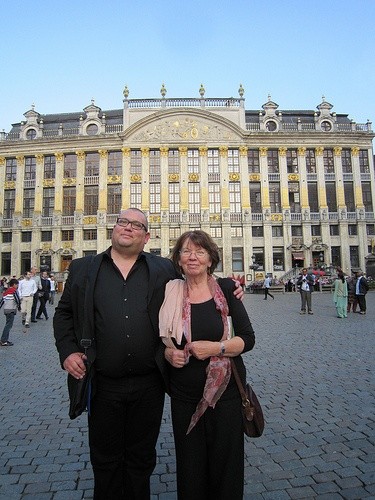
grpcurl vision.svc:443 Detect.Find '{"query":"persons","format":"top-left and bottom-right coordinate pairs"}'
top-left (262, 268), bottom-right (369, 318)
top-left (151, 230), bottom-right (255, 500)
top-left (0, 270), bottom-right (56, 346)
top-left (52, 208), bottom-right (244, 500)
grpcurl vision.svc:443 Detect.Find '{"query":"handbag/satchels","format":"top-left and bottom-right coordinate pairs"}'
top-left (67, 360), bottom-right (90, 420)
top-left (241, 381), bottom-right (265, 438)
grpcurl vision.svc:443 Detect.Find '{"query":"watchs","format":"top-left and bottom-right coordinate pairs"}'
top-left (220, 341), bottom-right (226, 358)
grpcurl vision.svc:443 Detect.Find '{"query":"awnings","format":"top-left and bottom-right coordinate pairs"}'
top-left (292, 254), bottom-right (305, 260)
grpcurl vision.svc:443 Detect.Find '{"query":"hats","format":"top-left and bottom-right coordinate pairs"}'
top-left (6, 279), bottom-right (19, 284)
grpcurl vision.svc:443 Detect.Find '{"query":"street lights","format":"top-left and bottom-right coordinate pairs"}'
top-left (251, 253), bottom-right (256, 281)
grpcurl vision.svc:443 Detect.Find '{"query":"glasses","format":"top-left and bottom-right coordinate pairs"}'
top-left (116, 216), bottom-right (148, 232)
top-left (179, 248), bottom-right (210, 257)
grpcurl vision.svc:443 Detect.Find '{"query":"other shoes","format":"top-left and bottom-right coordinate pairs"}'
top-left (45, 316), bottom-right (49, 320)
top-left (263, 298), bottom-right (267, 300)
top-left (31, 320), bottom-right (37, 323)
top-left (48, 300), bottom-right (54, 305)
top-left (24, 323), bottom-right (30, 328)
top-left (0, 341), bottom-right (14, 346)
top-left (359, 310), bottom-right (366, 314)
top-left (272, 296), bottom-right (274, 300)
top-left (353, 310), bottom-right (358, 313)
top-left (347, 310), bottom-right (350, 312)
top-left (300, 310), bottom-right (306, 314)
top-left (308, 310), bottom-right (313, 315)
top-left (337, 315), bottom-right (348, 318)
top-left (22, 320), bottom-right (25, 325)
top-left (36, 317), bottom-right (43, 320)
